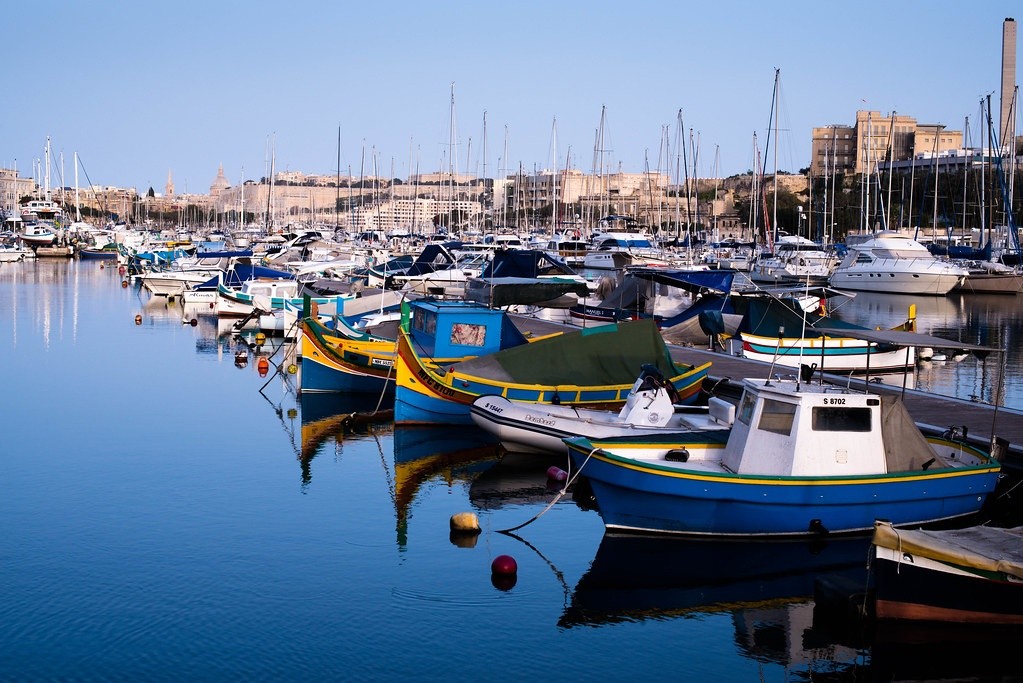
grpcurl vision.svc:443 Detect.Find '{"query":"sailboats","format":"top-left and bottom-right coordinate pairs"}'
top-left (750, 67), bottom-right (829, 281)
top-left (0, 83), bottom-right (1023, 330)
top-left (954, 91), bottom-right (1023, 294)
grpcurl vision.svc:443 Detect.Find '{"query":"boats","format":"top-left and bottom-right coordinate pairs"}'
top-left (392, 269), bottom-right (482, 295)
top-left (300, 298), bottom-right (564, 394)
top-left (394, 318), bottom-right (712, 424)
top-left (463, 249), bottom-right (617, 307)
top-left (829, 231), bottom-right (971, 296)
top-left (569, 265), bottom-right (821, 343)
top-left (560, 271), bottom-right (1001, 539)
top-left (740, 302), bottom-right (916, 372)
top-left (557, 532), bottom-right (871, 683)
top-left (469, 364), bottom-right (737, 462)
top-left (866, 520), bottom-right (1023, 625)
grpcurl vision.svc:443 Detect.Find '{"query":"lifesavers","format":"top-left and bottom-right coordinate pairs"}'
top-left (799, 259), bottom-right (805, 266)
top-left (21, 210), bottom-right (30, 214)
top-left (572, 229), bottom-right (582, 236)
top-left (427, 235), bottom-right (431, 242)
